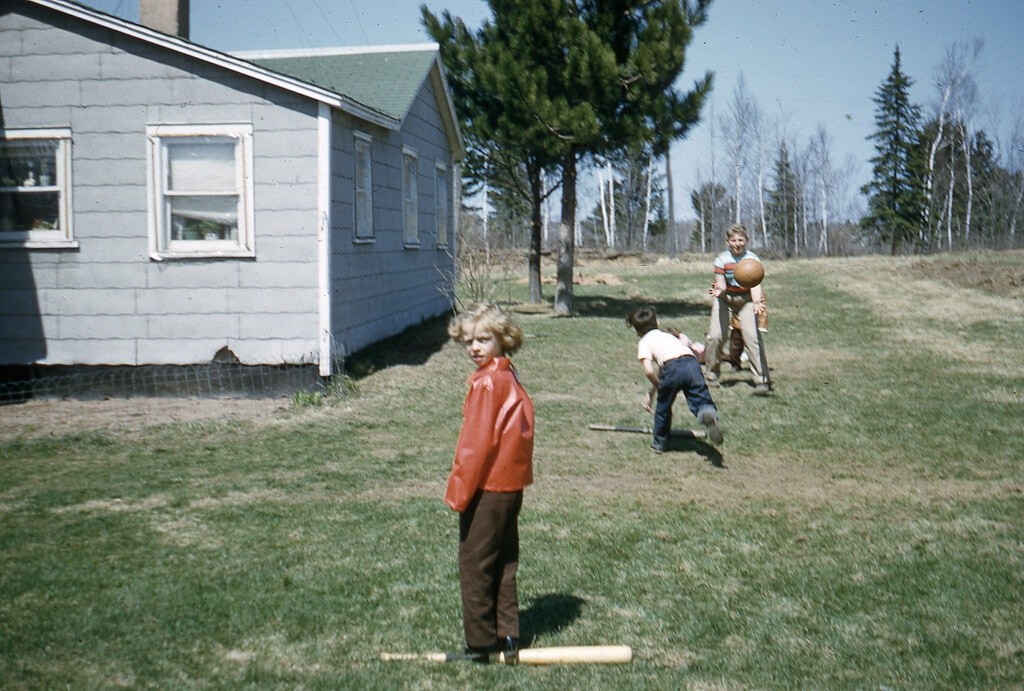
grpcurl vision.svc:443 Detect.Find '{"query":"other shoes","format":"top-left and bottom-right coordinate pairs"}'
top-left (702, 412), bottom-right (724, 445)
top-left (465, 648), bottom-right (488, 663)
top-left (757, 327), bottom-right (769, 332)
top-left (647, 442), bottom-right (663, 453)
top-left (753, 384), bottom-right (770, 396)
top-left (499, 637), bottom-right (518, 653)
top-left (733, 365), bottom-right (742, 372)
top-left (706, 372), bottom-right (717, 381)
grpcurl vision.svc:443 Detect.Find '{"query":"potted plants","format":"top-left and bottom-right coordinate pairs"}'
top-left (198, 221), bottom-right (223, 239)
top-left (39, 159), bottom-right (51, 186)
top-left (183, 224), bottom-right (200, 240)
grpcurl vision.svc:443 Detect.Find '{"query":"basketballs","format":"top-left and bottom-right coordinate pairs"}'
top-left (733, 258), bottom-right (764, 288)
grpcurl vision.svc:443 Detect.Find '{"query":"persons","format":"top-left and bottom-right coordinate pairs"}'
top-left (667, 290), bottom-right (769, 371)
top-left (445, 303), bottom-right (535, 660)
top-left (626, 305), bottom-right (723, 454)
top-left (706, 224), bottom-right (771, 396)
